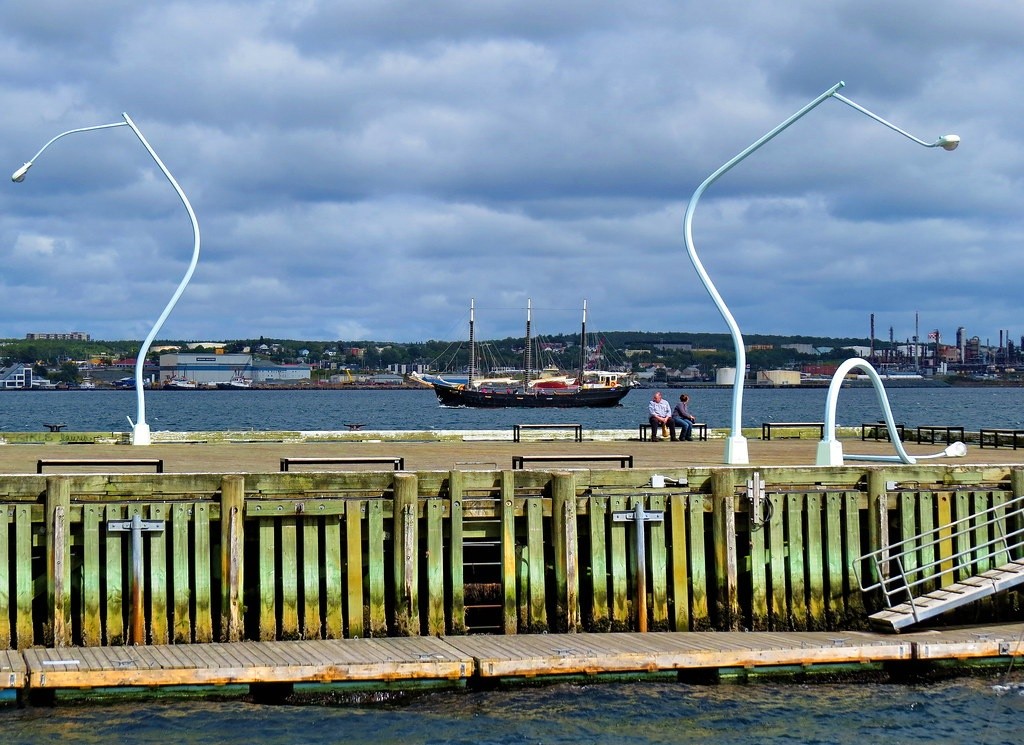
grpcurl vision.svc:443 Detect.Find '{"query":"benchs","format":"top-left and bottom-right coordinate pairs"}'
top-left (511, 453), bottom-right (634, 469)
top-left (37, 459), bottom-right (164, 474)
top-left (762, 422), bottom-right (825, 440)
top-left (979, 428), bottom-right (1024, 450)
top-left (917, 425), bottom-right (965, 445)
top-left (513, 423), bottom-right (582, 442)
top-left (639, 423), bottom-right (708, 442)
top-left (862, 423), bottom-right (904, 442)
top-left (280, 456), bottom-right (405, 472)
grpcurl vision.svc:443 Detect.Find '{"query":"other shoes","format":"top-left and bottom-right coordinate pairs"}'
top-left (671, 438), bottom-right (679, 441)
top-left (680, 438), bottom-right (685, 441)
top-left (686, 437), bottom-right (693, 441)
top-left (652, 437), bottom-right (659, 442)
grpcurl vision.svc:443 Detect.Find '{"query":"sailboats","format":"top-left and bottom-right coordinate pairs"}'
top-left (432, 298), bottom-right (632, 409)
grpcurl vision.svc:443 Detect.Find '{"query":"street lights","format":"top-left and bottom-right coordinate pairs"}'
top-left (684, 82), bottom-right (960, 467)
top-left (10, 111), bottom-right (201, 447)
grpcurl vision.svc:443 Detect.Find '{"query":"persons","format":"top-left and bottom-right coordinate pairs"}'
top-left (672, 394), bottom-right (696, 442)
top-left (647, 392), bottom-right (680, 443)
